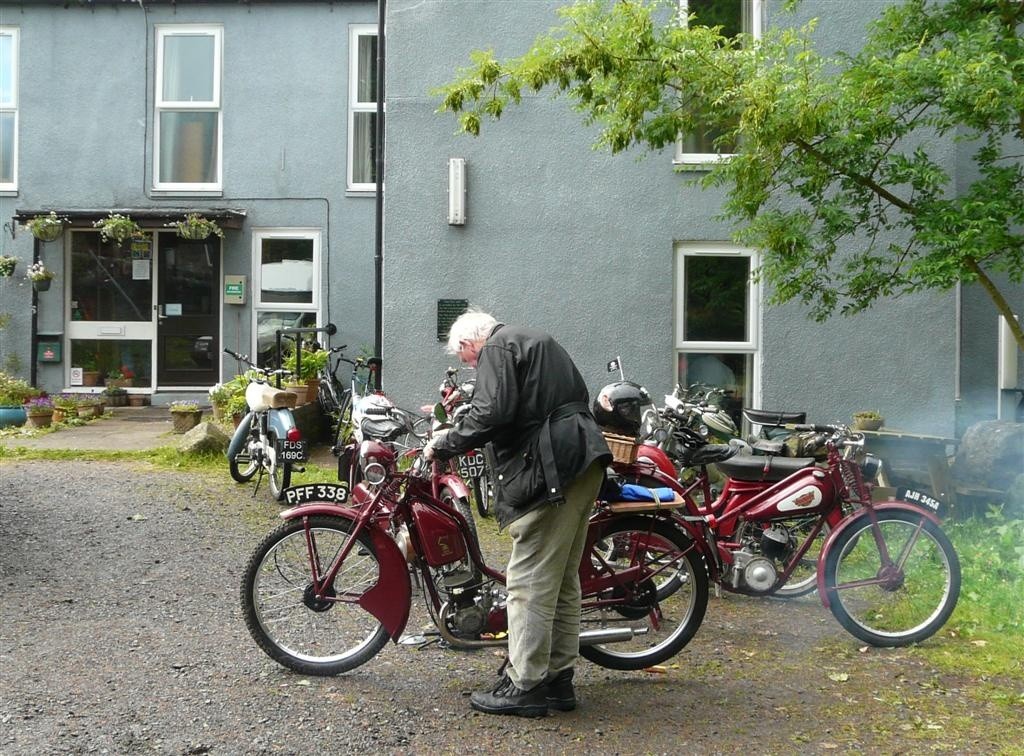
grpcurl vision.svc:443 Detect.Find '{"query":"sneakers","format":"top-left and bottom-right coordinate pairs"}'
top-left (469, 673), bottom-right (547, 717)
top-left (534, 668), bottom-right (576, 712)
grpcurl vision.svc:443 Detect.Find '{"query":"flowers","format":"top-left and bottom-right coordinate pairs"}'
top-left (91, 212), bottom-right (144, 249)
top-left (167, 399), bottom-right (200, 411)
top-left (0, 256), bottom-right (57, 287)
top-left (24, 211), bottom-right (72, 242)
top-left (30, 398), bottom-right (54, 413)
top-left (163, 212), bottom-right (228, 241)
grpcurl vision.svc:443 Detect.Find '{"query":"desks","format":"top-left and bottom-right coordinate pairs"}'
top-left (856, 429), bottom-right (961, 507)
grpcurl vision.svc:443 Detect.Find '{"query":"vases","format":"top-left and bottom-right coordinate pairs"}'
top-left (39, 224), bottom-right (64, 241)
top-left (33, 277), bottom-right (53, 291)
top-left (128, 394), bottom-right (146, 406)
top-left (1, 263), bottom-right (16, 276)
top-left (30, 413), bottom-right (53, 429)
top-left (172, 410), bottom-right (204, 434)
top-left (180, 223), bottom-right (213, 240)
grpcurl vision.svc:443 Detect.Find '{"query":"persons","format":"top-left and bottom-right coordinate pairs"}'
top-left (688, 353), bottom-right (734, 410)
top-left (420, 307), bottom-right (613, 713)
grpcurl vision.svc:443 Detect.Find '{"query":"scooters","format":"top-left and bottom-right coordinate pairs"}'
top-left (236, 392), bottom-right (731, 675)
top-left (318, 344), bottom-right (867, 605)
top-left (636, 422), bottom-right (963, 650)
top-left (219, 345), bottom-right (310, 500)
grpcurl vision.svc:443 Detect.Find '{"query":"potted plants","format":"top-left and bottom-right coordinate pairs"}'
top-left (80, 354), bottom-right (124, 386)
top-left (855, 412), bottom-right (884, 431)
top-left (209, 376), bottom-right (249, 431)
top-left (0, 373), bottom-right (40, 430)
top-left (281, 343), bottom-right (328, 405)
top-left (51, 386), bottom-right (127, 430)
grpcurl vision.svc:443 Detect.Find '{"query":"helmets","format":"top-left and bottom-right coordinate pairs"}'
top-left (593, 380), bottom-right (652, 433)
